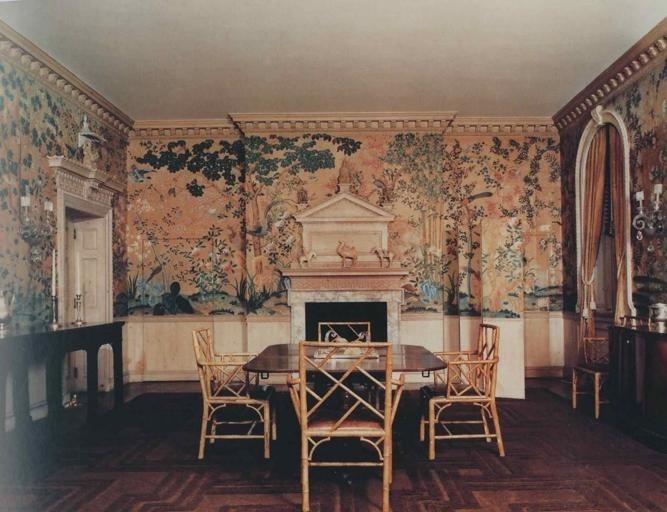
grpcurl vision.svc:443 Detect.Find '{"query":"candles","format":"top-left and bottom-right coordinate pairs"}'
top-left (52, 249), bottom-right (56, 297)
top-left (76, 251), bottom-right (81, 294)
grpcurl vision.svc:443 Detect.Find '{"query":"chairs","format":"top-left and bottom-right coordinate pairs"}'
top-left (419, 323), bottom-right (505, 460)
top-left (192, 327), bottom-right (277, 459)
top-left (318, 322), bottom-right (380, 412)
top-left (571, 337), bottom-right (610, 419)
top-left (287, 341), bottom-right (405, 512)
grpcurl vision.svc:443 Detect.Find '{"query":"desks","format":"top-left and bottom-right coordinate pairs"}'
top-left (241, 342), bottom-right (448, 485)
top-left (0, 321), bottom-right (124, 432)
top-left (608, 324), bottom-right (667, 455)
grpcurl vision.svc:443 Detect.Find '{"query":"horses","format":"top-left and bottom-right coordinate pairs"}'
top-left (298, 251), bottom-right (317, 267)
top-left (371, 246), bottom-right (395, 268)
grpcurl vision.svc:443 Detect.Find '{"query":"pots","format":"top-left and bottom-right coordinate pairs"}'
top-left (649, 304), bottom-right (667, 321)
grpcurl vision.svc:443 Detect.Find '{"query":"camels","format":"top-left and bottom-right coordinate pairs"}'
top-left (337, 240), bottom-right (358, 267)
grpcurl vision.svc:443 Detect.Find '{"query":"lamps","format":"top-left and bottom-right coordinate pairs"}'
top-left (19, 196), bottom-right (56, 267)
top-left (632, 184), bottom-right (667, 252)
